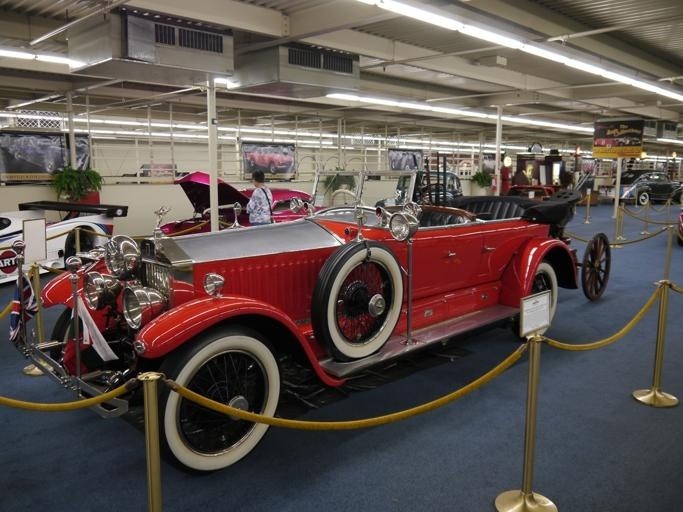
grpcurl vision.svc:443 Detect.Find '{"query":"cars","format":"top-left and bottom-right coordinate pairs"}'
top-left (612, 138), bottom-right (640, 147)
top-left (676, 209), bottom-right (683, 246)
top-left (377, 172), bottom-right (462, 207)
top-left (609, 169), bottom-right (682, 205)
top-left (4, 170), bottom-right (582, 475)
top-left (244, 145), bottom-right (294, 175)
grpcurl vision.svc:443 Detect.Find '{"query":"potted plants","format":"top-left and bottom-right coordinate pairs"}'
top-left (50, 167), bottom-right (104, 205)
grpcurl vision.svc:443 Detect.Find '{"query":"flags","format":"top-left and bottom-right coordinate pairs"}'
top-left (9, 265), bottom-right (41, 342)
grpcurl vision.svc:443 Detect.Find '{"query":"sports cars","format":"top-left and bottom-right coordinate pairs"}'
top-left (152, 171), bottom-right (328, 237)
top-left (0, 200), bottom-right (128, 285)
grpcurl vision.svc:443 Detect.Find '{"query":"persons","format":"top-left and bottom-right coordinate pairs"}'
top-left (244, 166), bottom-right (275, 227)
top-left (521, 161), bottom-right (539, 185)
